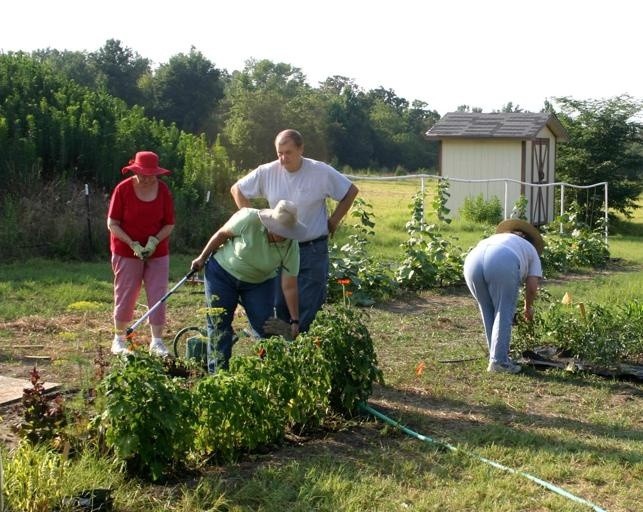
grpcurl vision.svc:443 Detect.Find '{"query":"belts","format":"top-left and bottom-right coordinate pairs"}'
top-left (297, 235), bottom-right (328, 249)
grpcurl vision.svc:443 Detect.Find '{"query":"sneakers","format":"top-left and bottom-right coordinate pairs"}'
top-left (148, 343), bottom-right (172, 358)
top-left (487, 361), bottom-right (521, 374)
top-left (504, 357), bottom-right (512, 364)
top-left (111, 335), bottom-right (127, 355)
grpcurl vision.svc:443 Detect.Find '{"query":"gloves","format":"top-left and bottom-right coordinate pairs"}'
top-left (133, 236), bottom-right (159, 261)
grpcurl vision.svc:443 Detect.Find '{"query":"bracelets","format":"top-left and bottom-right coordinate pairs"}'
top-left (289, 317), bottom-right (298, 324)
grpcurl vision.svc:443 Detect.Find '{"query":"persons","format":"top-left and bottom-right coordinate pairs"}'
top-left (106, 150), bottom-right (176, 357)
top-left (229, 129), bottom-right (360, 332)
top-left (461, 227), bottom-right (543, 374)
top-left (191, 200), bottom-right (304, 373)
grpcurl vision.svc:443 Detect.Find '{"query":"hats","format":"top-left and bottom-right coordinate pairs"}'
top-left (121, 150), bottom-right (170, 176)
top-left (496, 219), bottom-right (543, 257)
top-left (258, 199), bottom-right (308, 240)
